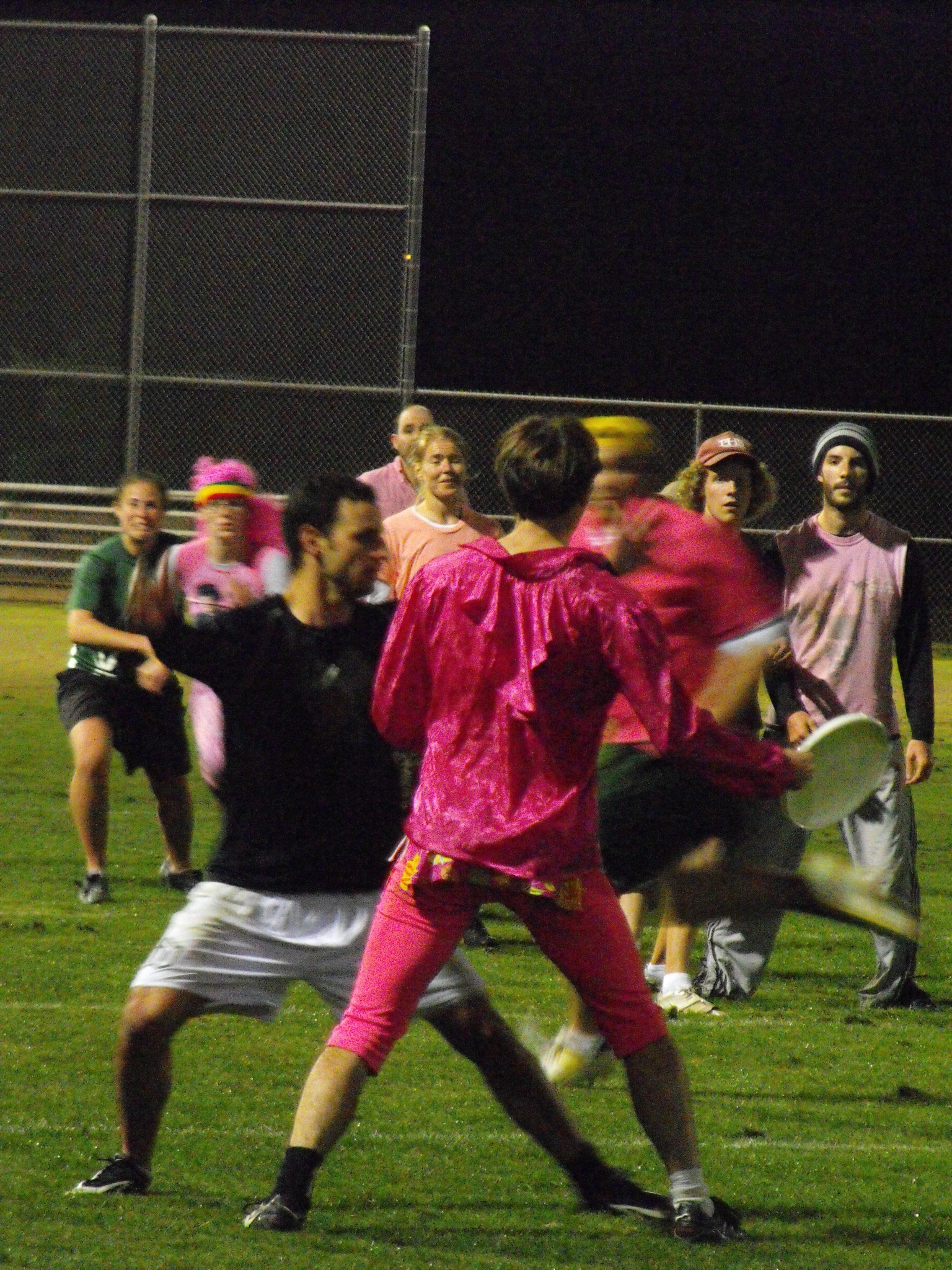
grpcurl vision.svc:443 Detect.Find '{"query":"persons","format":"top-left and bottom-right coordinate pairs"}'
top-left (55, 404), bottom-right (936, 1085)
top-left (244, 417), bottom-right (743, 1245)
top-left (71, 476), bottom-right (671, 1223)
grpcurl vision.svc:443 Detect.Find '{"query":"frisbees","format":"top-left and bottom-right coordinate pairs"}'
top-left (786, 712), bottom-right (889, 831)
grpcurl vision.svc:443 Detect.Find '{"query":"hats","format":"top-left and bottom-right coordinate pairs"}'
top-left (695, 430), bottom-right (759, 468)
top-left (650, 481), bottom-right (681, 503)
top-left (578, 417), bottom-right (653, 455)
top-left (812, 423), bottom-right (879, 494)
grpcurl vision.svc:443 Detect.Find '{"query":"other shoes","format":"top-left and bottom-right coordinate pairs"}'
top-left (539, 1024), bottom-right (613, 1089)
top-left (80, 874), bottom-right (111, 904)
top-left (459, 915), bottom-right (502, 953)
top-left (161, 855), bottom-right (201, 893)
top-left (690, 957), bottom-right (747, 1004)
top-left (891, 973), bottom-right (935, 1012)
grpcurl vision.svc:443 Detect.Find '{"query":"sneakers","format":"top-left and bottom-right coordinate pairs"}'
top-left (669, 1195), bottom-right (748, 1245)
top-left (599, 1175), bottom-right (672, 1227)
top-left (644, 968), bottom-right (663, 992)
top-left (241, 1196), bottom-right (311, 1232)
top-left (655, 985), bottom-right (727, 1020)
top-left (63, 1154), bottom-right (151, 1197)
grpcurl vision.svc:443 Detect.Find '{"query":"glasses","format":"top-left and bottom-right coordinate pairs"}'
top-left (204, 499), bottom-right (244, 510)
